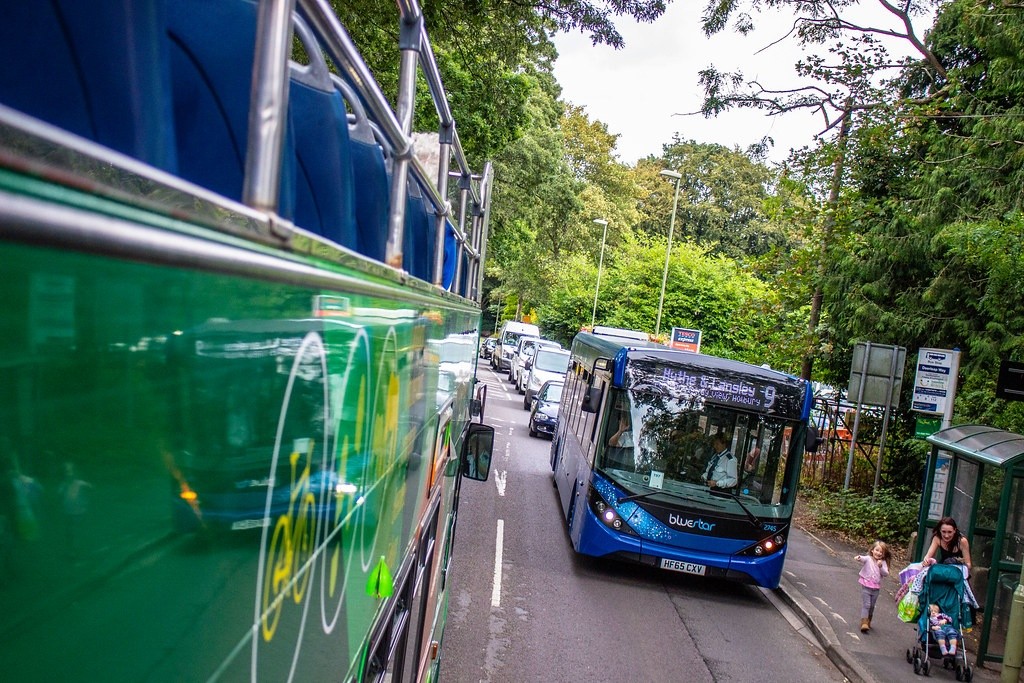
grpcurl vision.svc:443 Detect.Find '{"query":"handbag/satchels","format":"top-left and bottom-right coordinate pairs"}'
top-left (894, 562), bottom-right (928, 623)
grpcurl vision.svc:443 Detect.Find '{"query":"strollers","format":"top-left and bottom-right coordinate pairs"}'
top-left (907, 557), bottom-right (974, 683)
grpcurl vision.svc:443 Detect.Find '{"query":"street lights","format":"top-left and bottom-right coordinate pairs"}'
top-left (653, 168), bottom-right (683, 336)
top-left (590, 218), bottom-right (609, 328)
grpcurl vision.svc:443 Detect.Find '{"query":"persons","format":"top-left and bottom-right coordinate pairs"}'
top-left (922, 517), bottom-right (973, 656)
top-left (467, 436), bottom-right (489, 480)
top-left (609, 412), bottom-right (738, 499)
top-left (854, 540), bottom-right (891, 631)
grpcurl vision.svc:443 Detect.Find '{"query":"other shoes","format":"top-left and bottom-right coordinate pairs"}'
top-left (861, 616), bottom-right (873, 631)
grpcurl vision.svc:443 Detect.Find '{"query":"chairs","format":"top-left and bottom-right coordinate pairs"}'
top-left (0, 0), bottom-right (469, 296)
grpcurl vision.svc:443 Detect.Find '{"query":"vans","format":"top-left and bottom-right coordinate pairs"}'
top-left (492, 320), bottom-right (540, 373)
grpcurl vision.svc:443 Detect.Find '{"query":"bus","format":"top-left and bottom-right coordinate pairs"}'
top-left (549, 325), bottom-right (825, 588)
top-left (0, 1), bottom-right (496, 683)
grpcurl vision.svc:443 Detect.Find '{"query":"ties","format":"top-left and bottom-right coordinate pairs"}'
top-left (707, 457), bottom-right (720, 480)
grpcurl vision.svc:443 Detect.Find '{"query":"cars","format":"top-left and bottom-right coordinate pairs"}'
top-left (480, 338), bottom-right (498, 360)
top-left (528, 379), bottom-right (564, 442)
top-left (515, 344), bottom-right (538, 394)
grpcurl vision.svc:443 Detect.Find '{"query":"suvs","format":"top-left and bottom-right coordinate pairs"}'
top-left (509, 336), bottom-right (561, 382)
top-left (524, 344), bottom-right (571, 410)
